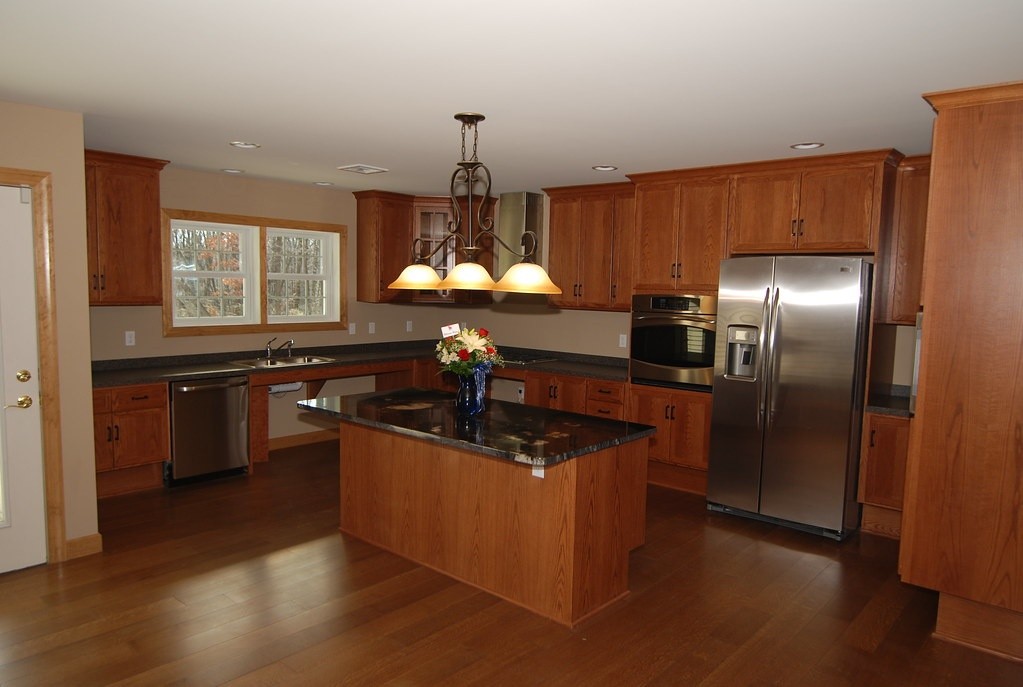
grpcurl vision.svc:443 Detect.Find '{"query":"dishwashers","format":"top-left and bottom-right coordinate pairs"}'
top-left (168, 376), bottom-right (251, 481)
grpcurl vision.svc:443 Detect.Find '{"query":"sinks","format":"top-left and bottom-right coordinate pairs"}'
top-left (245, 360), bottom-right (287, 368)
top-left (285, 356), bottom-right (332, 363)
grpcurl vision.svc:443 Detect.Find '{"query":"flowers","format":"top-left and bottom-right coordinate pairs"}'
top-left (436, 326), bottom-right (501, 371)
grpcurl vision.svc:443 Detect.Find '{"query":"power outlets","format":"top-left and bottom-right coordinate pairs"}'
top-left (406, 321), bottom-right (413, 333)
top-left (369, 321), bottom-right (375, 334)
top-left (349, 323), bottom-right (356, 335)
top-left (125, 329), bottom-right (134, 346)
top-left (532, 465), bottom-right (545, 479)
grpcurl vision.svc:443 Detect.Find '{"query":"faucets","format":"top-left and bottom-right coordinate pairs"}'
top-left (266, 338), bottom-right (294, 359)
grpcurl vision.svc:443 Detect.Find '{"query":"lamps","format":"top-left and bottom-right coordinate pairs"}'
top-left (388, 110), bottom-right (563, 295)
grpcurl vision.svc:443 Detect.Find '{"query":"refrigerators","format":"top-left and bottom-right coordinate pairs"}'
top-left (701, 254), bottom-right (874, 544)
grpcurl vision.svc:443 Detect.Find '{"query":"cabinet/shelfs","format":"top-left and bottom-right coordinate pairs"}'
top-left (858, 413), bottom-right (909, 511)
top-left (883, 161), bottom-right (934, 325)
top-left (84, 147), bottom-right (172, 307)
top-left (632, 384), bottom-right (709, 467)
top-left (635, 178), bottom-right (730, 294)
top-left (587, 380), bottom-right (627, 420)
top-left (609, 191), bottom-right (637, 311)
top-left (95, 382), bottom-right (173, 471)
top-left (554, 195), bottom-right (614, 311)
top-left (728, 165), bottom-right (881, 254)
top-left (527, 373), bottom-right (586, 412)
top-left (352, 189), bottom-right (499, 303)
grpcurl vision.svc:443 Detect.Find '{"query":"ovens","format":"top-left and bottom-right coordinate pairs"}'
top-left (628, 291), bottom-right (718, 392)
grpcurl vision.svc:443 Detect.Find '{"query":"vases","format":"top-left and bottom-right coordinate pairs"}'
top-left (453, 365), bottom-right (484, 416)
